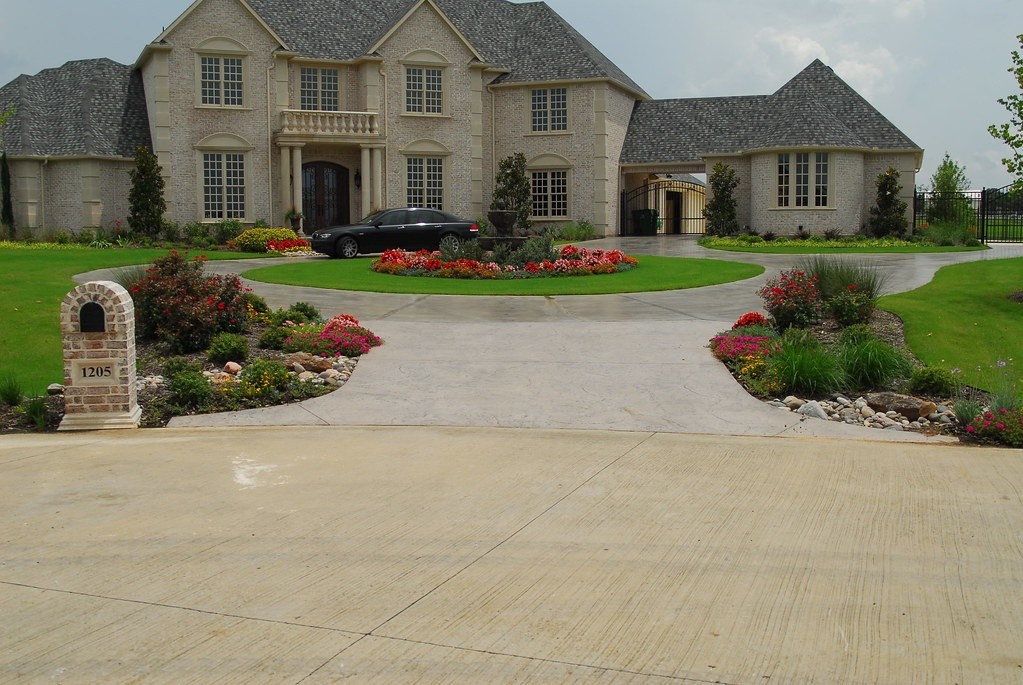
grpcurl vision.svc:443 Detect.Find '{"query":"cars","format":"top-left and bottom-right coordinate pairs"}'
top-left (310, 206), bottom-right (480, 260)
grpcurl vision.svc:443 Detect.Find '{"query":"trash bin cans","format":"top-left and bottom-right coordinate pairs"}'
top-left (631, 209), bottom-right (658, 236)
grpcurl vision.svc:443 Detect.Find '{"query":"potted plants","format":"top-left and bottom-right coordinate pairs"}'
top-left (284, 207), bottom-right (305, 237)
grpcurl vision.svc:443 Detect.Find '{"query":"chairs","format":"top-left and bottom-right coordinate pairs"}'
top-left (389, 213), bottom-right (398, 224)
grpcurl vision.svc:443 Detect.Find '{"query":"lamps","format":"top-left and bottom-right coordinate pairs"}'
top-left (290, 174), bottom-right (293, 185)
top-left (354, 168), bottom-right (361, 189)
